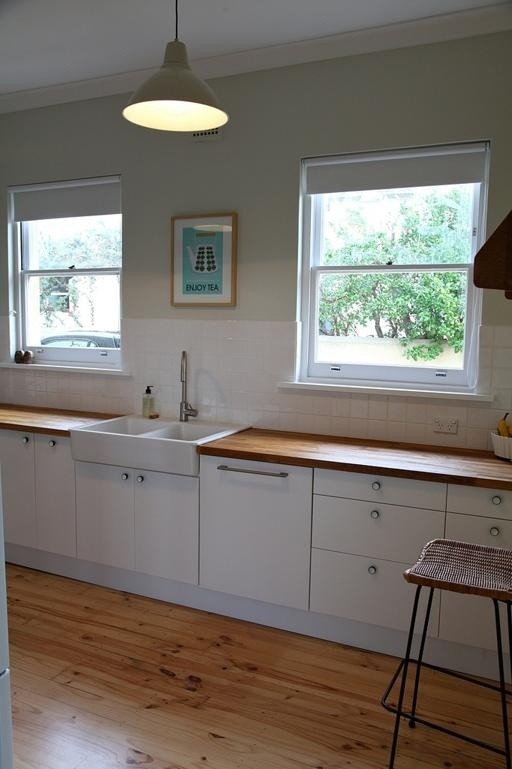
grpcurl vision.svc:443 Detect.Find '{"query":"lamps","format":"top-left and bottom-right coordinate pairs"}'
top-left (120, 0), bottom-right (231, 132)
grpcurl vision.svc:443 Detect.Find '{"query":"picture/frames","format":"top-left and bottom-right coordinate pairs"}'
top-left (169, 211), bottom-right (238, 307)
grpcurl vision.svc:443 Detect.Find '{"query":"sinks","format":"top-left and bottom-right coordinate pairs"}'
top-left (139, 422), bottom-right (252, 477)
top-left (69, 414), bottom-right (169, 467)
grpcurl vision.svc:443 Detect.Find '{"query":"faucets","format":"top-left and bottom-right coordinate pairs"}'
top-left (180, 350), bottom-right (197, 422)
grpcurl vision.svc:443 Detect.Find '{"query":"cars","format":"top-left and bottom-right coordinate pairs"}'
top-left (40, 329), bottom-right (121, 348)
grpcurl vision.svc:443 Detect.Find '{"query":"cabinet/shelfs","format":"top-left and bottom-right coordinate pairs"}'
top-left (74, 460), bottom-right (200, 588)
top-left (1, 427), bottom-right (76, 560)
top-left (310, 465), bottom-right (447, 639)
top-left (439, 481), bottom-right (512, 652)
top-left (198, 452), bottom-right (313, 612)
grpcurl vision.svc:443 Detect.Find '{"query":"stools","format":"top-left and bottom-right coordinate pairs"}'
top-left (381, 537), bottom-right (512, 769)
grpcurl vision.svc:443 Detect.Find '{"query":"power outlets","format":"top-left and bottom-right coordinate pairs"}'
top-left (431, 415), bottom-right (458, 434)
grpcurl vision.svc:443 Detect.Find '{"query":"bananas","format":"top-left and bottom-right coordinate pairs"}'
top-left (496, 413), bottom-right (512, 438)
